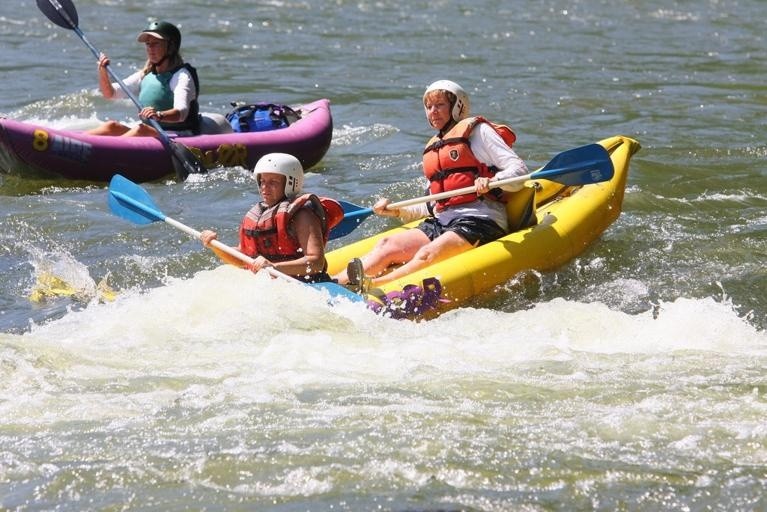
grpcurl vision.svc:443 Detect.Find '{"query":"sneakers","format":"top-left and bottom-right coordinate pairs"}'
top-left (344, 257), bottom-right (372, 298)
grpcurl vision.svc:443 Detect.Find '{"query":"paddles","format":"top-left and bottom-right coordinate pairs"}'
top-left (327, 145), bottom-right (615, 241)
top-left (38, 0), bottom-right (206, 180)
top-left (108, 174), bottom-right (364, 314)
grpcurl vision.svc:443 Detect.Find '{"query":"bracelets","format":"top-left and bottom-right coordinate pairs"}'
top-left (156, 110), bottom-right (163, 122)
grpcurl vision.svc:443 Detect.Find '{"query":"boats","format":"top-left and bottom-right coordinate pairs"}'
top-left (27, 134), bottom-right (645, 324)
top-left (0, 97), bottom-right (335, 184)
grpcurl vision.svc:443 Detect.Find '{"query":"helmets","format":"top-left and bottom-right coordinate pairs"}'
top-left (253, 152), bottom-right (304, 200)
top-left (423, 78), bottom-right (470, 131)
top-left (137, 19), bottom-right (182, 58)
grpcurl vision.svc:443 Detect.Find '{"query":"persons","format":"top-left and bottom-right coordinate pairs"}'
top-left (200, 153), bottom-right (334, 283)
top-left (329, 80), bottom-right (529, 296)
top-left (86, 19), bottom-right (208, 139)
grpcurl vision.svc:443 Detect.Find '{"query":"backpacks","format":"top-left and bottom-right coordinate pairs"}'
top-left (225, 102), bottom-right (298, 131)
